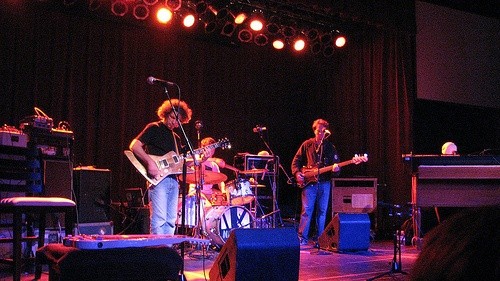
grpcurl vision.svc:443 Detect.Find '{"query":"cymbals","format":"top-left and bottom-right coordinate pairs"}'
top-left (239, 169), bottom-right (270, 174)
top-left (249, 185), bottom-right (266, 188)
top-left (179, 169), bottom-right (228, 185)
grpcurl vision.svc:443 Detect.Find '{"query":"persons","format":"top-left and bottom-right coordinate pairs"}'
top-left (187, 137), bottom-right (225, 195)
top-left (129, 99), bottom-right (213, 247)
top-left (407, 207), bottom-right (500, 281)
top-left (291, 118), bottom-right (341, 246)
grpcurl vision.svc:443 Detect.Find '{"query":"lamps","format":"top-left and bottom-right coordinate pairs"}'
top-left (90, 0), bottom-right (347, 58)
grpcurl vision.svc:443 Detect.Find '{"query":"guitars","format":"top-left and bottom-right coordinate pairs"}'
top-left (63, 235), bottom-right (212, 250)
top-left (122, 136), bottom-right (232, 187)
top-left (296, 153), bottom-right (368, 188)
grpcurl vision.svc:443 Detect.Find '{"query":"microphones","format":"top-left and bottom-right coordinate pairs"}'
top-left (253, 127), bottom-right (266, 132)
top-left (147, 76), bottom-right (173, 88)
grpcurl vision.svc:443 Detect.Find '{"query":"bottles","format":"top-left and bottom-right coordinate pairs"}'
top-left (399, 230), bottom-right (405, 247)
top-left (395, 230), bottom-right (399, 247)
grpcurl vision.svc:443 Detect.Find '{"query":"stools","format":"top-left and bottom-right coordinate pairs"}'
top-left (0, 198), bottom-right (76, 281)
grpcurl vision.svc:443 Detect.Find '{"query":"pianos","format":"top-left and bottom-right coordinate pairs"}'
top-left (401, 151), bottom-right (500, 250)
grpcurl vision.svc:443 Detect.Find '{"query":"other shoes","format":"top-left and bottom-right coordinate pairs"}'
top-left (300, 239), bottom-right (309, 245)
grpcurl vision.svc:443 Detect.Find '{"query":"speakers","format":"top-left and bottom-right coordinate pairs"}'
top-left (73, 167), bottom-right (110, 224)
top-left (318, 213), bottom-right (370, 252)
top-left (209, 226), bottom-right (300, 281)
top-left (39, 241), bottom-right (188, 281)
top-left (37, 159), bottom-right (73, 227)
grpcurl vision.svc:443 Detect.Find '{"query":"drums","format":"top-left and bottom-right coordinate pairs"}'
top-left (202, 205), bottom-right (256, 249)
top-left (175, 194), bottom-right (197, 229)
top-left (224, 178), bottom-right (254, 206)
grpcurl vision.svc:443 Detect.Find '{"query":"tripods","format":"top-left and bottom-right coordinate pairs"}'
top-left (161, 90), bottom-right (286, 259)
